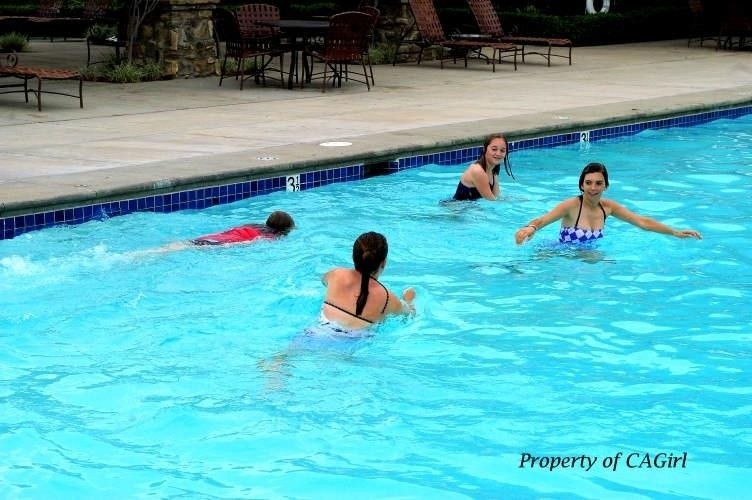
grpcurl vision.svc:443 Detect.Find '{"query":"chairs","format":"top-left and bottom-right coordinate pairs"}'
top-left (392, 0), bottom-right (573, 72)
top-left (211, 4), bottom-right (381, 93)
top-left (0, 54), bottom-right (84, 111)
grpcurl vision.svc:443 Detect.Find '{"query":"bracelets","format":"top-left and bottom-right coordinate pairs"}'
top-left (526, 223), bottom-right (539, 230)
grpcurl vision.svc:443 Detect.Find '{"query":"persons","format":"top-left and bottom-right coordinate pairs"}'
top-left (454, 133), bottom-right (515, 201)
top-left (122, 210), bottom-right (297, 257)
top-left (513, 160), bottom-right (703, 265)
top-left (266, 230), bottom-right (417, 393)
